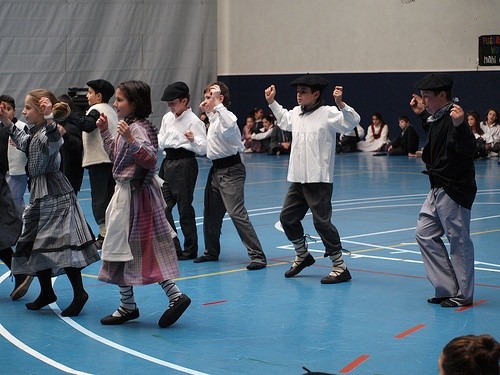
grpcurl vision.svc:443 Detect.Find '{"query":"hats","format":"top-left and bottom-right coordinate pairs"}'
top-left (413, 72), bottom-right (454, 89)
top-left (289, 75), bottom-right (330, 90)
top-left (161, 82), bottom-right (189, 101)
top-left (86, 80), bottom-right (115, 96)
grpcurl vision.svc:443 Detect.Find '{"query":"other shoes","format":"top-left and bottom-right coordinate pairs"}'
top-left (177, 250), bottom-right (196, 261)
top-left (101, 308), bottom-right (140, 325)
top-left (285, 253), bottom-right (316, 278)
top-left (9, 275), bottom-right (35, 300)
top-left (321, 267), bottom-right (351, 283)
top-left (159, 293), bottom-right (191, 327)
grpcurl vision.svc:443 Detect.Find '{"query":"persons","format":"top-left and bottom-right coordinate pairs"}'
top-left (0, 80), bottom-right (210, 317)
top-left (335, 113), bottom-right (430, 158)
top-left (95, 80), bottom-right (191, 328)
top-left (439, 334), bottom-right (500, 375)
top-left (193, 82), bottom-right (267, 269)
top-left (240, 108), bottom-right (292, 155)
top-left (408, 72), bottom-right (477, 306)
top-left (263, 73), bottom-right (361, 284)
top-left (466, 108), bottom-right (500, 160)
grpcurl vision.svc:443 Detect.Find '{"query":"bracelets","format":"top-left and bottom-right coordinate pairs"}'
top-left (43, 113), bottom-right (54, 119)
